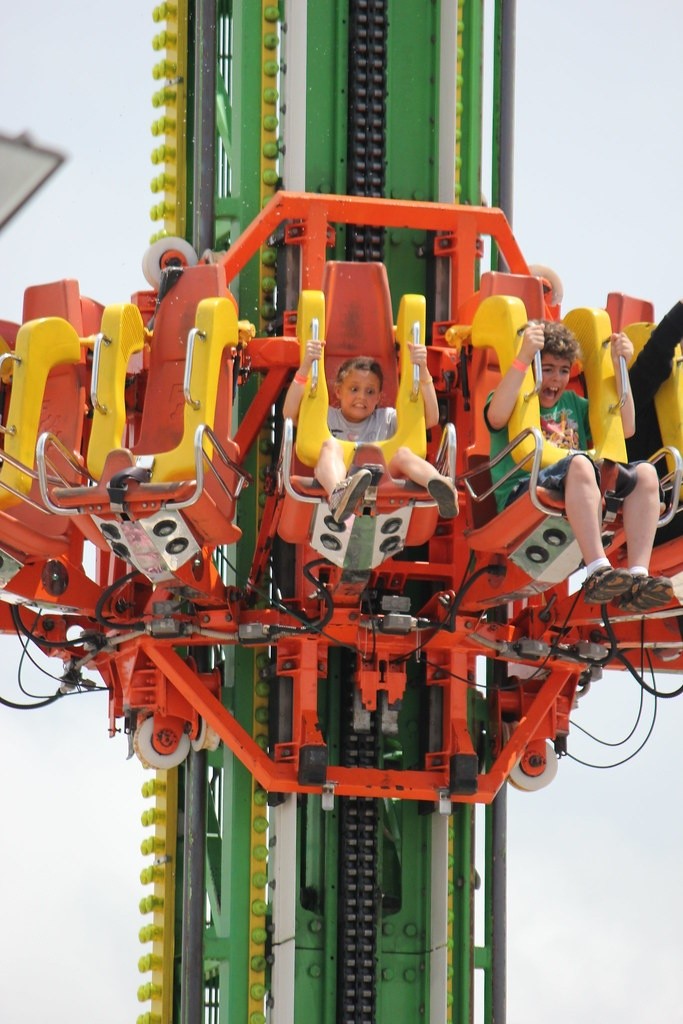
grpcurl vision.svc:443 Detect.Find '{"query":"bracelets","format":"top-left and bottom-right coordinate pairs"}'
top-left (292, 371), bottom-right (308, 384)
top-left (419, 375), bottom-right (433, 385)
top-left (512, 355), bottom-right (529, 373)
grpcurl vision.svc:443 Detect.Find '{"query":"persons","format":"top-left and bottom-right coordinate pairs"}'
top-left (484, 319), bottom-right (673, 612)
top-left (282, 339), bottom-right (459, 524)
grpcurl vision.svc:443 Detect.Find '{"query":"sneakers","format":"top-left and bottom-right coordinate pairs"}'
top-left (583, 567), bottom-right (633, 604)
top-left (617, 572), bottom-right (673, 612)
top-left (326, 468), bottom-right (371, 523)
top-left (427, 478), bottom-right (460, 518)
